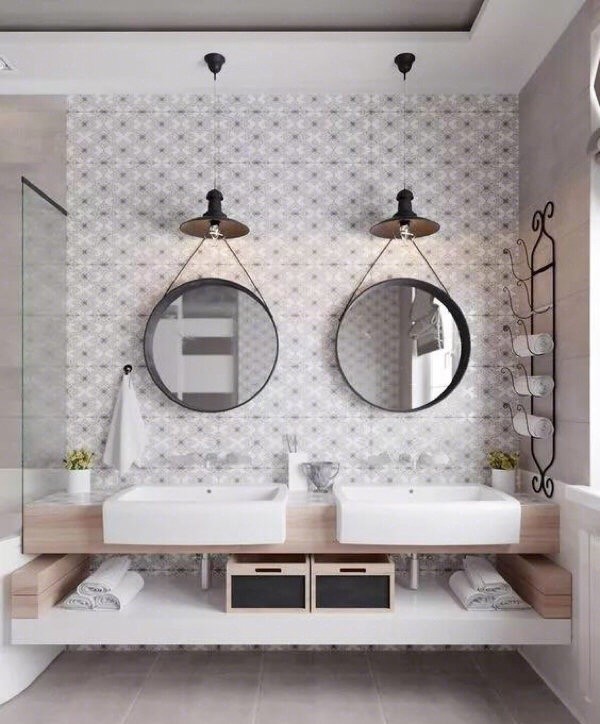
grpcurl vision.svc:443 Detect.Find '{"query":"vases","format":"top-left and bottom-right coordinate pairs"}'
top-left (491, 469), bottom-right (516, 494)
top-left (68, 470), bottom-right (90, 493)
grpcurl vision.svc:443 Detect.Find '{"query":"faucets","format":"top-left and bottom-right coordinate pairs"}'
top-left (205, 453), bottom-right (218, 471)
top-left (399, 453), bottom-right (414, 469)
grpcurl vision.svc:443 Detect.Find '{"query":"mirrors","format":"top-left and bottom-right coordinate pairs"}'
top-left (336, 278), bottom-right (471, 414)
top-left (143, 278), bottom-right (280, 413)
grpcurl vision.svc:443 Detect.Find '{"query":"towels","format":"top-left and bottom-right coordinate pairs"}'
top-left (512, 413), bottom-right (555, 440)
top-left (64, 556), bottom-right (144, 610)
top-left (512, 375), bottom-right (555, 397)
top-left (103, 372), bottom-right (148, 475)
top-left (448, 556), bottom-right (531, 612)
top-left (512, 333), bottom-right (554, 358)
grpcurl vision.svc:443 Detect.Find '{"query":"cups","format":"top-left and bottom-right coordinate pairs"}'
top-left (302, 462), bottom-right (341, 494)
top-left (288, 452), bottom-right (307, 493)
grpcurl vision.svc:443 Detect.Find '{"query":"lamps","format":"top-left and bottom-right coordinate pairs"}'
top-left (179, 53), bottom-right (250, 241)
top-left (369, 53), bottom-right (441, 240)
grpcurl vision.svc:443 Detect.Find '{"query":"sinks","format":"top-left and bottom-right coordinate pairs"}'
top-left (100, 481), bottom-right (290, 547)
top-left (331, 483), bottom-right (522, 549)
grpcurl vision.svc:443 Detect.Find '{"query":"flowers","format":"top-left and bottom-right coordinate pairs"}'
top-left (61, 449), bottom-right (93, 469)
top-left (487, 451), bottom-right (518, 469)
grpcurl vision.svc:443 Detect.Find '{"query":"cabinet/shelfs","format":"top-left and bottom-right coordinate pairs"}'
top-left (502, 201), bottom-right (555, 498)
top-left (8, 502), bottom-right (572, 645)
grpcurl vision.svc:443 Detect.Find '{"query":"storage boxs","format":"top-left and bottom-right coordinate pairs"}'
top-left (311, 554), bottom-right (395, 613)
top-left (226, 553), bottom-right (310, 612)
